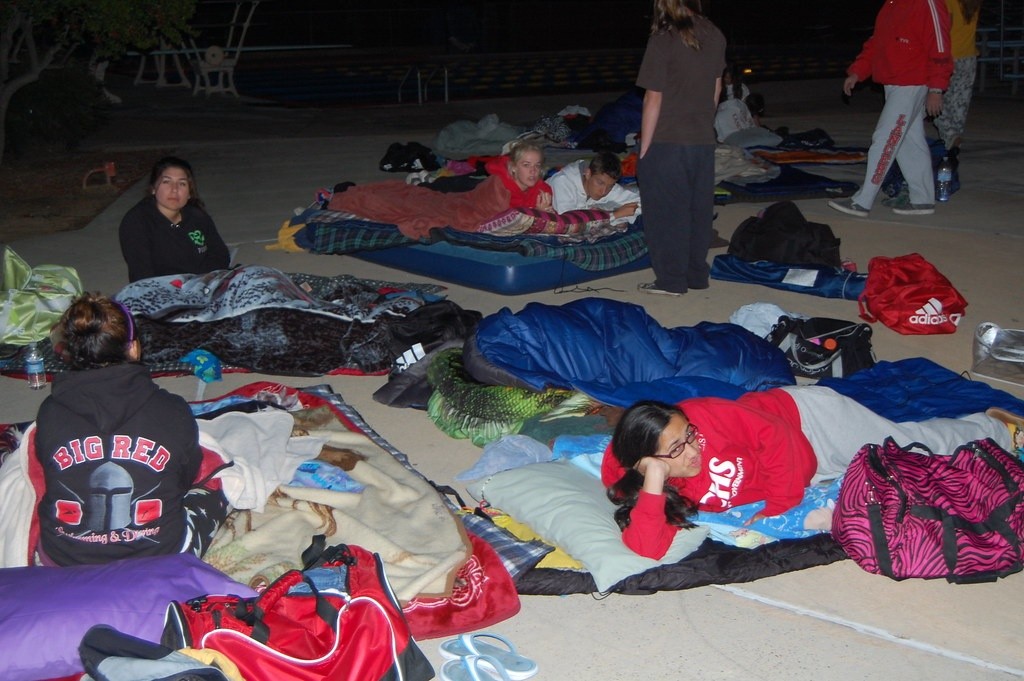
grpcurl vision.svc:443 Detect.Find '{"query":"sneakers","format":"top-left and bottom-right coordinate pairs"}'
top-left (638, 283), bottom-right (682, 296)
top-left (893, 204), bottom-right (935, 214)
top-left (827, 198), bottom-right (869, 217)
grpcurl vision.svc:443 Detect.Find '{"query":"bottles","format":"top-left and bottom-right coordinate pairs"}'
top-left (935, 157), bottom-right (952, 201)
top-left (25, 342), bottom-right (48, 389)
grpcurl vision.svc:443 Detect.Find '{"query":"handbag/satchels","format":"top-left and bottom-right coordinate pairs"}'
top-left (727, 200), bottom-right (840, 276)
top-left (76, 535), bottom-right (436, 681)
top-left (380, 142), bottom-right (440, 171)
top-left (972, 322), bottom-right (1024, 387)
top-left (0, 245), bottom-right (83, 359)
top-left (763, 315), bottom-right (877, 379)
top-left (858, 253), bottom-right (969, 335)
top-left (831, 435), bottom-right (1024, 584)
top-left (882, 139), bottom-right (961, 197)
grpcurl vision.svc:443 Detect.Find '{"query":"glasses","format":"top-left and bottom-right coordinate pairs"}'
top-left (650, 423), bottom-right (698, 459)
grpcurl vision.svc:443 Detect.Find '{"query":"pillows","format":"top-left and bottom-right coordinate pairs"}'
top-left (467, 456), bottom-right (713, 593)
top-left (0, 552), bottom-right (259, 681)
top-left (723, 128), bottom-right (782, 148)
top-left (476, 207), bottom-right (609, 240)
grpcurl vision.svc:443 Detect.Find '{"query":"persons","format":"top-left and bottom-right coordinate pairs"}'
top-left (560, 91), bottom-right (646, 150)
top-left (634, 0), bottom-right (727, 296)
top-left (827, 0), bottom-right (980, 217)
top-left (601, 386), bottom-right (1024, 561)
top-left (119, 157), bottom-right (230, 283)
top-left (318, 137), bottom-right (558, 217)
top-left (713, 68), bottom-right (764, 144)
top-left (33, 288), bottom-right (232, 567)
top-left (405, 153), bottom-right (642, 231)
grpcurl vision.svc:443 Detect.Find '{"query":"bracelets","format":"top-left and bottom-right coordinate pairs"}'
top-left (928, 89), bottom-right (943, 93)
